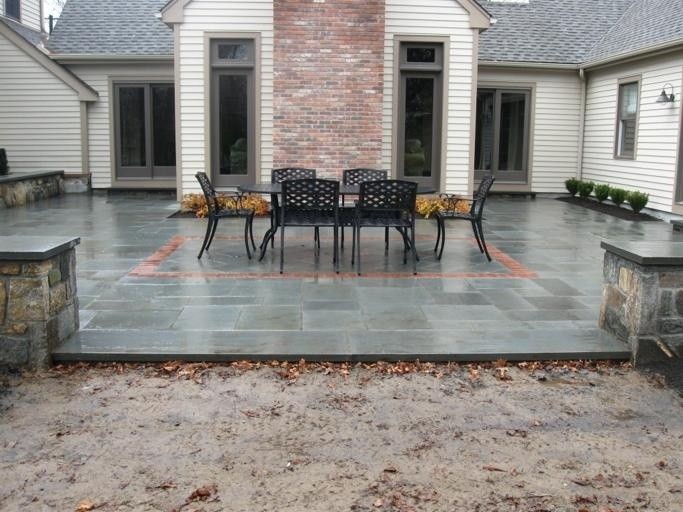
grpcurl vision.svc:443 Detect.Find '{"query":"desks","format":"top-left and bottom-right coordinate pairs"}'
top-left (238, 182), bottom-right (437, 262)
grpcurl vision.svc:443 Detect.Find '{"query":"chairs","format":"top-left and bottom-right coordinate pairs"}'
top-left (433, 174), bottom-right (495, 263)
top-left (194, 171), bottom-right (256, 259)
top-left (270, 167), bottom-right (418, 277)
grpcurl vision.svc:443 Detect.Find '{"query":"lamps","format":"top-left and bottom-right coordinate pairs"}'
top-left (656, 82), bottom-right (674, 104)
